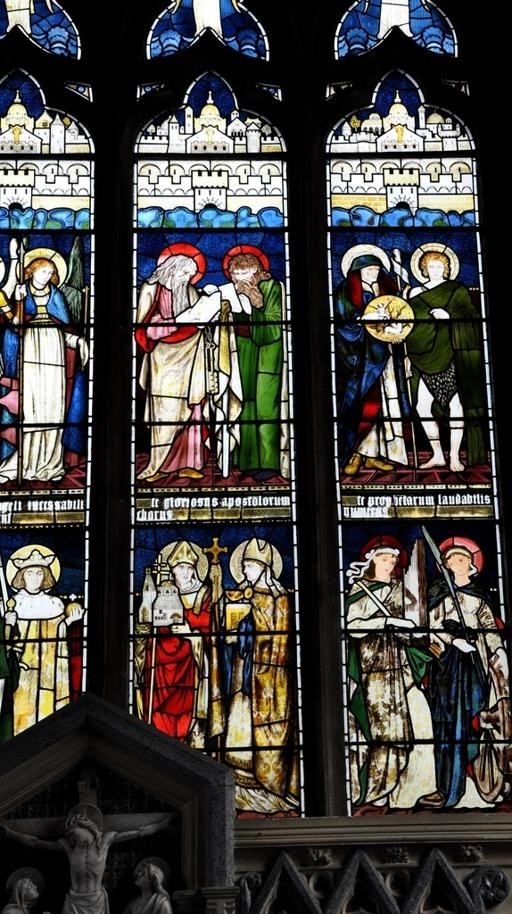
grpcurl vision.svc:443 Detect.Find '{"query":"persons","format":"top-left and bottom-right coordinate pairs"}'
top-left (1, 879), bottom-right (51, 914)
top-left (0, 815), bottom-right (176, 914)
top-left (123, 864), bottom-right (173, 914)
